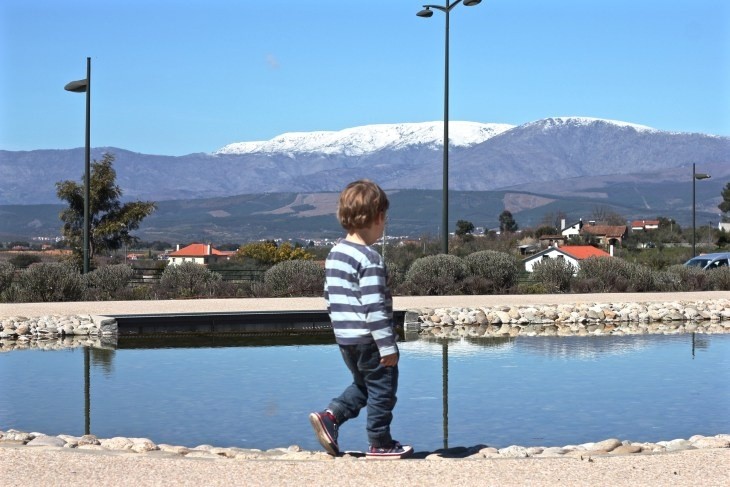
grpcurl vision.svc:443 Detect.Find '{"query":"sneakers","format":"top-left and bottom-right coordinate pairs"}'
top-left (309, 408), bottom-right (341, 457)
top-left (366, 440), bottom-right (414, 459)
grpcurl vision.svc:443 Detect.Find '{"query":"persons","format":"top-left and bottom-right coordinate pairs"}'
top-left (309, 179), bottom-right (414, 460)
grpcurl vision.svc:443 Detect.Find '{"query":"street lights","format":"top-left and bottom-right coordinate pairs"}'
top-left (64, 79), bottom-right (89, 275)
top-left (416, 0), bottom-right (481, 255)
top-left (692, 174), bottom-right (711, 258)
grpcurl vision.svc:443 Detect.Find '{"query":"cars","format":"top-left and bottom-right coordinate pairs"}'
top-left (683, 252), bottom-right (730, 271)
